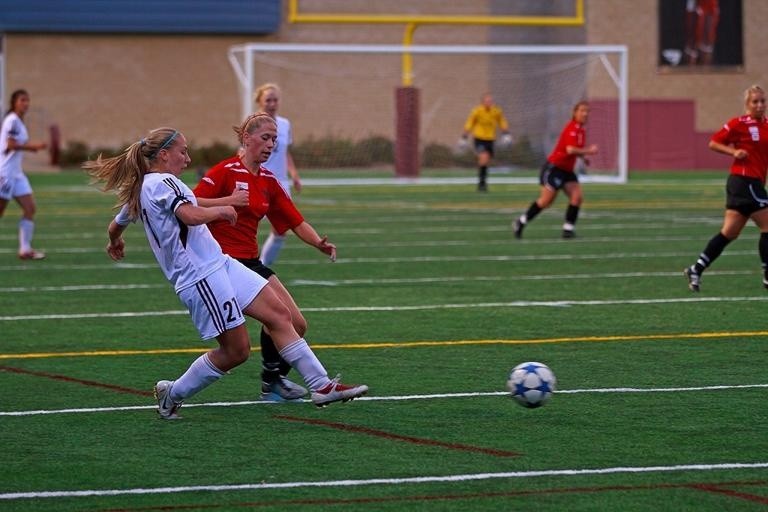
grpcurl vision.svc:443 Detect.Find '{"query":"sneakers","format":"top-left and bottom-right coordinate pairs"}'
top-left (513, 217), bottom-right (525, 237)
top-left (154, 380), bottom-right (181, 421)
top-left (562, 227), bottom-right (575, 240)
top-left (18, 246), bottom-right (45, 260)
top-left (684, 264), bottom-right (700, 293)
top-left (310, 373), bottom-right (370, 407)
top-left (261, 374), bottom-right (308, 399)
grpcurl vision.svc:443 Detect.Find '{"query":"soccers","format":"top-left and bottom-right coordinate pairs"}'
top-left (509, 361), bottom-right (556, 407)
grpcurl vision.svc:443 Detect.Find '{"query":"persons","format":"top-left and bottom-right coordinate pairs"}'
top-left (683, 1), bottom-right (721, 69)
top-left (81, 126), bottom-right (369, 419)
top-left (0, 89), bottom-right (48, 259)
top-left (684, 84), bottom-right (768, 293)
top-left (459, 90), bottom-right (514, 191)
top-left (192, 112), bottom-right (337, 402)
top-left (236, 81), bottom-right (302, 268)
top-left (512, 100), bottom-right (598, 240)
